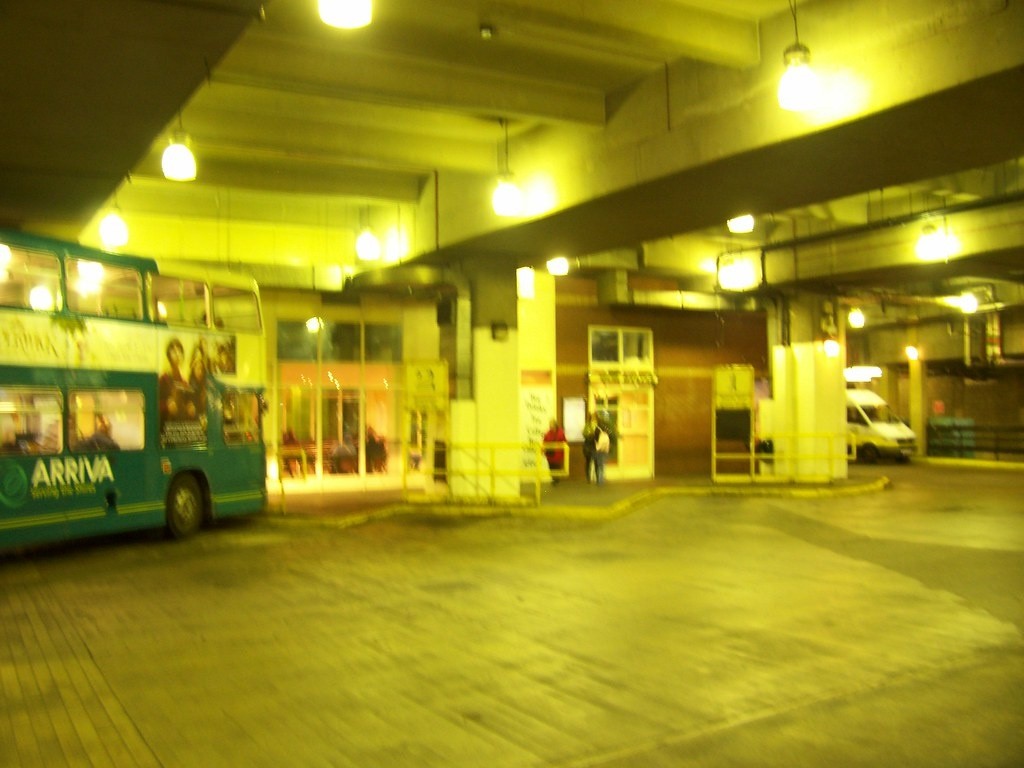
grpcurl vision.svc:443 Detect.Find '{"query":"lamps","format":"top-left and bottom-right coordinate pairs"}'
top-left (160, 114), bottom-right (196, 183)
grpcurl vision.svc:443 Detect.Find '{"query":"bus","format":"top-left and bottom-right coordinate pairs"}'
top-left (1, 228), bottom-right (269, 560)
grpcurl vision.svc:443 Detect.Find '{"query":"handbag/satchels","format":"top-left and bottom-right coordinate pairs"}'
top-left (549, 451), bottom-right (555, 458)
top-left (596, 432), bottom-right (610, 452)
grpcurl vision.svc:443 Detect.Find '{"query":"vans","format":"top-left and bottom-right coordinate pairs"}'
top-left (846, 388), bottom-right (918, 465)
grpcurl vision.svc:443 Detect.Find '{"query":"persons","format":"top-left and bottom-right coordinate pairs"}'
top-left (283, 412), bottom-right (387, 479)
top-left (583, 411), bottom-right (610, 486)
top-left (161, 335), bottom-right (243, 446)
top-left (543, 420), bottom-right (566, 486)
